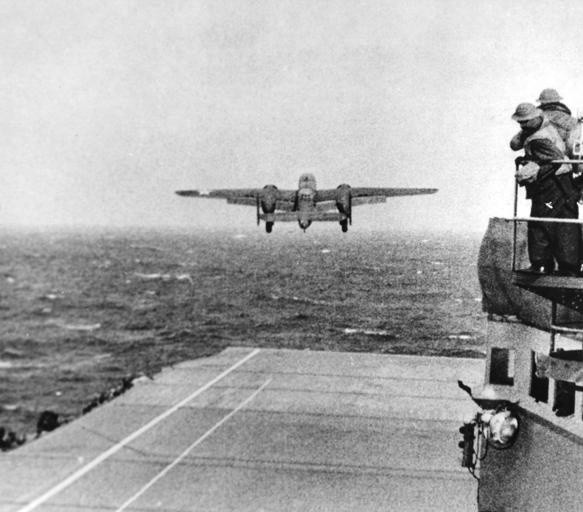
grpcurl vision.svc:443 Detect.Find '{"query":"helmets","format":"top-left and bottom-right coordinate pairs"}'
top-left (512, 103), bottom-right (542, 121)
top-left (536, 90), bottom-right (560, 107)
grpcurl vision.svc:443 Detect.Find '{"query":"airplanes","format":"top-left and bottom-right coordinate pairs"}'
top-left (176, 174), bottom-right (438, 233)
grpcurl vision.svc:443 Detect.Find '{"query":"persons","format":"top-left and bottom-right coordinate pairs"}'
top-left (508, 102), bottom-right (582, 276)
top-left (511, 89), bottom-right (583, 203)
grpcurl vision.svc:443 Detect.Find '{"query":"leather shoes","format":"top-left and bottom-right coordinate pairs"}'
top-left (518, 260), bottom-right (555, 273)
top-left (556, 268), bottom-right (583, 276)
